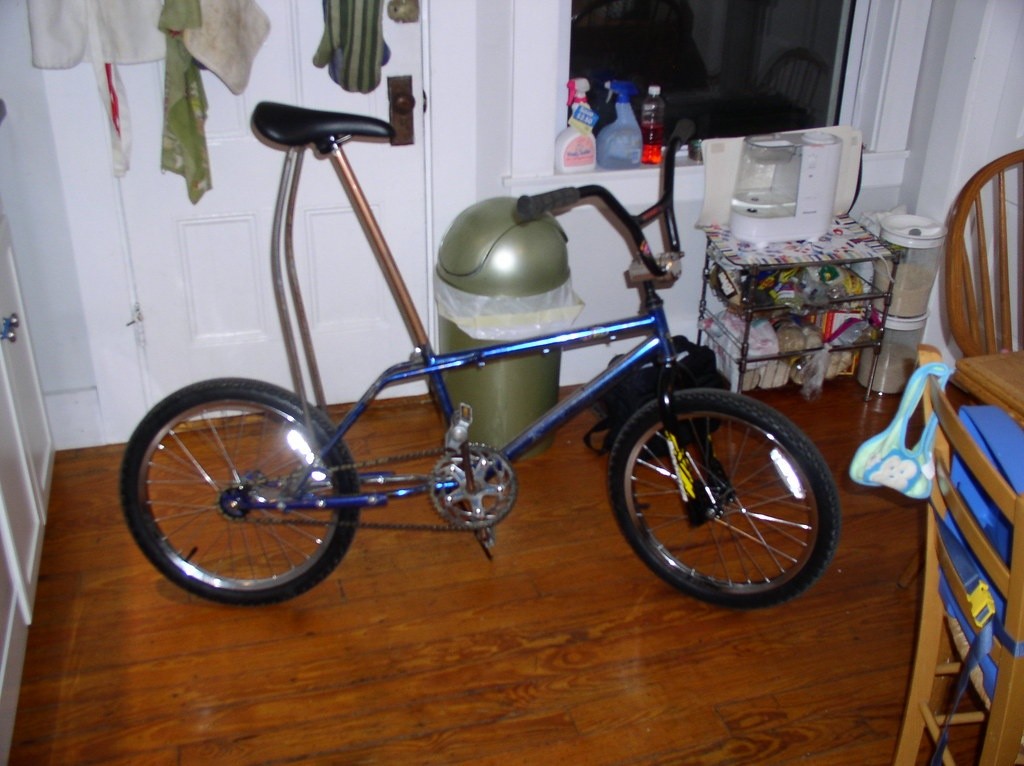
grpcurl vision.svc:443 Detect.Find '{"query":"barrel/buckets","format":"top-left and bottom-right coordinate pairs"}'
top-left (873, 214), bottom-right (948, 320)
top-left (857, 313), bottom-right (931, 394)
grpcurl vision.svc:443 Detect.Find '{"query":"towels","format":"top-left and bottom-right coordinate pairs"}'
top-left (25, 0), bottom-right (270, 206)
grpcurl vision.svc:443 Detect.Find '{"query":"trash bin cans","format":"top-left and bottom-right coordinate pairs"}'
top-left (433, 196), bottom-right (570, 460)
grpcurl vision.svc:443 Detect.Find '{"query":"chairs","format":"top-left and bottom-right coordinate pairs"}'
top-left (893, 342), bottom-right (1024, 766)
top-left (942, 147), bottom-right (1024, 360)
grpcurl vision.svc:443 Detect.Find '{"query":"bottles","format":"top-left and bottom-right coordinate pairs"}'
top-left (640, 86), bottom-right (666, 164)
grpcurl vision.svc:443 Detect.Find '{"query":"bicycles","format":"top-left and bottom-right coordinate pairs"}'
top-left (120, 101), bottom-right (841, 608)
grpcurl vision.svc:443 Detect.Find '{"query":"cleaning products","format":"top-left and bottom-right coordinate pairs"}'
top-left (555, 77), bottom-right (597, 173)
top-left (597, 79), bottom-right (643, 170)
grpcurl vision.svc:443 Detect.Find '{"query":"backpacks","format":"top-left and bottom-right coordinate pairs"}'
top-left (583, 333), bottom-right (730, 459)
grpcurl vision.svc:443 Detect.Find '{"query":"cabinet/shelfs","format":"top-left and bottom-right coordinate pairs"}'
top-left (697, 215), bottom-right (904, 401)
top-left (0, 210), bottom-right (55, 766)
